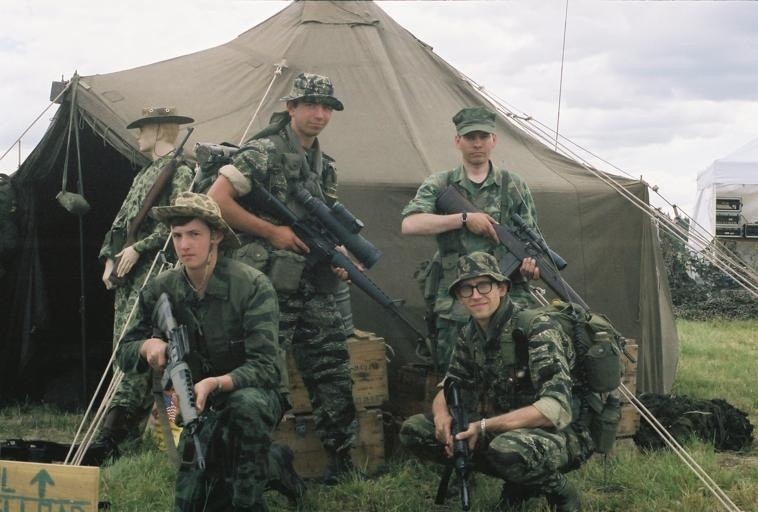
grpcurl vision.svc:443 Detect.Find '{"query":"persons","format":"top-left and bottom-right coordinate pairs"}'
top-left (400, 107), bottom-right (546, 372)
top-left (95, 103), bottom-right (198, 447)
top-left (192, 72), bottom-right (367, 483)
top-left (114, 192), bottom-right (305, 512)
top-left (399, 249), bottom-right (596, 512)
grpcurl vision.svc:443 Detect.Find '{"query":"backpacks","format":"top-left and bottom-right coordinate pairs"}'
top-left (189, 135), bottom-right (241, 194)
top-left (465, 299), bottom-right (623, 456)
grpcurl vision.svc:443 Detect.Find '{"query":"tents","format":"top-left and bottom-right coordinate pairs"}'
top-left (9, 0), bottom-right (678, 457)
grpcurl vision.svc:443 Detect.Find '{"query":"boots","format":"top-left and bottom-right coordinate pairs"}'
top-left (322, 451), bottom-right (380, 485)
top-left (552, 475), bottom-right (583, 511)
top-left (496, 477), bottom-right (541, 510)
top-left (268, 442), bottom-right (306, 510)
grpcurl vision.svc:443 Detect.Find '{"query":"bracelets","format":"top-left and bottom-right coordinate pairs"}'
top-left (460, 210), bottom-right (469, 227)
top-left (212, 376), bottom-right (224, 398)
top-left (480, 417), bottom-right (486, 439)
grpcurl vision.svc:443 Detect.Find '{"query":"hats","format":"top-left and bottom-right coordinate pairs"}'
top-left (279, 70), bottom-right (345, 111)
top-left (149, 190), bottom-right (244, 250)
top-left (448, 252), bottom-right (513, 300)
top-left (125, 107), bottom-right (196, 130)
top-left (452, 106), bottom-right (498, 137)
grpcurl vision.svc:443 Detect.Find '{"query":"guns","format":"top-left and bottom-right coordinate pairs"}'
top-left (105, 127), bottom-right (195, 287)
top-left (435, 377), bottom-right (468, 509)
top-left (436, 184), bottom-right (637, 364)
top-left (242, 177), bottom-right (426, 343)
top-left (150, 291), bottom-right (208, 474)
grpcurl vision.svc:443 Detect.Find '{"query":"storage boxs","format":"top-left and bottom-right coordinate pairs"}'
top-left (388, 334), bottom-right (641, 476)
top-left (281, 326), bottom-right (389, 415)
top-left (269, 406), bottom-right (387, 488)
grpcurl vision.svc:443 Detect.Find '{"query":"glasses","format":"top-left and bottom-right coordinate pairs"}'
top-left (453, 280), bottom-right (499, 299)
